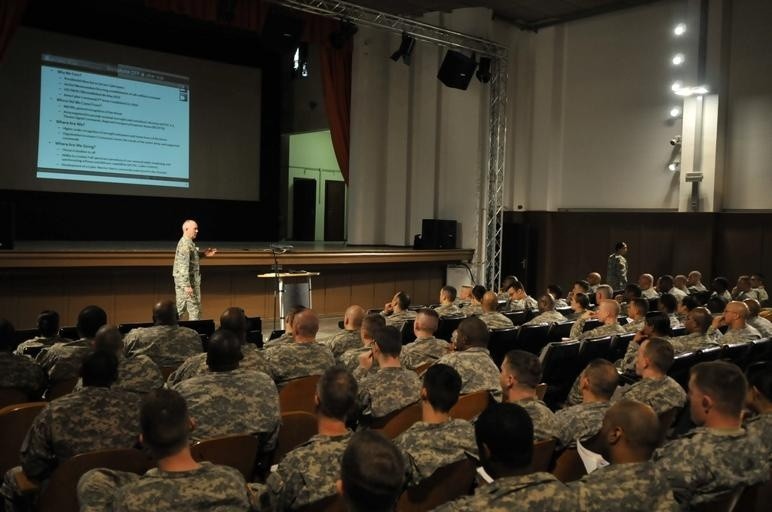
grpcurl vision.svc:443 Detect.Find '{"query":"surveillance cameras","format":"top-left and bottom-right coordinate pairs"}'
top-left (670, 134), bottom-right (681, 147)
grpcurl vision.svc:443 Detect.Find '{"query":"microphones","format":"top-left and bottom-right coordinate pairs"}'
top-left (272, 249), bottom-right (279, 277)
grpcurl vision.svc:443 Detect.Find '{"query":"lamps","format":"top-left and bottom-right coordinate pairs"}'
top-left (323, 15), bottom-right (495, 87)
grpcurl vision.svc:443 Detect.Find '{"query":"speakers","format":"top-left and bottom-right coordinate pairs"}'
top-left (422, 216), bottom-right (458, 250)
top-left (0, 201), bottom-right (19, 250)
top-left (435, 49), bottom-right (476, 91)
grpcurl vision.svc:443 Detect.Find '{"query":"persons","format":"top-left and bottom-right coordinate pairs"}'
top-left (0, 348), bottom-right (143, 511)
top-left (3, 272), bottom-right (771, 451)
top-left (172, 217), bottom-right (218, 323)
top-left (430, 401), bottom-right (577, 511)
top-left (605, 238), bottom-right (631, 289)
top-left (389, 362), bottom-right (479, 488)
top-left (647, 359), bottom-right (768, 506)
top-left (71, 386), bottom-right (256, 511)
top-left (578, 398), bottom-right (678, 510)
top-left (333, 425), bottom-right (409, 511)
top-left (247, 364), bottom-right (360, 510)
